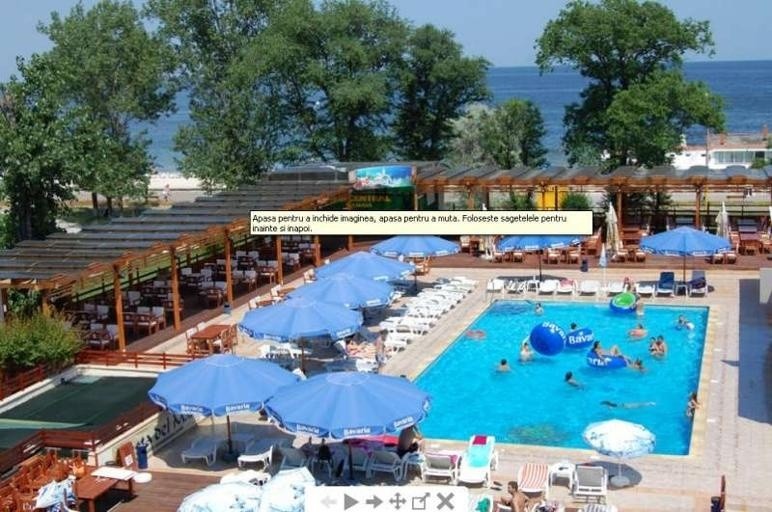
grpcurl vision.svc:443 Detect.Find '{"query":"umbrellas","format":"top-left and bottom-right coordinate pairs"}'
top-left (147, 353), bottom-right (299, 453)
top-left (285, 272), bottom-right (397, 310)
top-left (261, 369), bottom-right (430, 482)
top-left (581, 419), bottom-right (656, 479)
top-left (238, 295), bottom-right (364, 372)
top-left (314, 250), bottom-right (416, 283)
top-left (370, 235), bottom-right (461, 265)
top-left (496, 235), bottom-right (586, 281)
top-left (639, 225), bottom-right (732, 283)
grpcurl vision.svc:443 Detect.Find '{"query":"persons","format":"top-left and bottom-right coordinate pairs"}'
top-left (495, 275), bottom-right (701, 422)
top-left (397, 425), bottom-right (422, 459)
top-left (507, 480), bottom-right (529, 512)
top-left (346, 338), bottom-right (363, 354)
top-left (375, 331), bottom-right (386, 367)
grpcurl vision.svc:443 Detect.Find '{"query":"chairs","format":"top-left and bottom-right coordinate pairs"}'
top-left (66, 229), bottom-right (476, 378)
top-left (1, 433), bottom-right (618, 512)
top-left (476, 227), bottom-right (770, 299)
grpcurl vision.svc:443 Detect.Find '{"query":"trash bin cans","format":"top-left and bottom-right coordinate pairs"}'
top-left (224, 303), bottom-right (231, 313)
top-left (582, 259), bottom-right (588, 271)
top-left (138, 446), bottom-right (148, 468)
